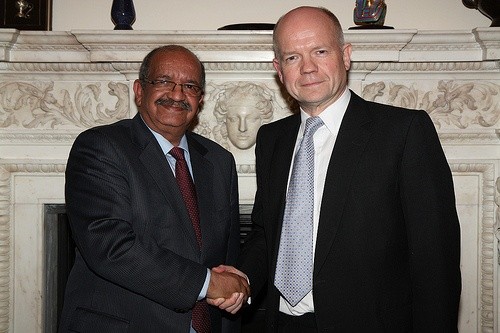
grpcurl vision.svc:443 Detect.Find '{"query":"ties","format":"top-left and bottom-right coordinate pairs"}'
top-left (274, 118), bottom-right (322, 307)
top-left (171, 147), bottom-right (213, 333)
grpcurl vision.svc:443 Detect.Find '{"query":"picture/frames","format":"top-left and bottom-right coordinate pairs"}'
top-left (0, 0), bottom-right (52, 31)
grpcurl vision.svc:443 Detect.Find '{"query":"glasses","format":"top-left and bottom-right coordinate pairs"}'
top-left (144, 78), bottom-right (200, 92)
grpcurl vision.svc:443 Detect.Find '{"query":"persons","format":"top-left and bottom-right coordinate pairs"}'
top-left (206, 6), bottom-right (463, 333)
top-left (58, 45), bottom-right (252, 333)
top-left (214, 83), bottom-right (273, 150)
